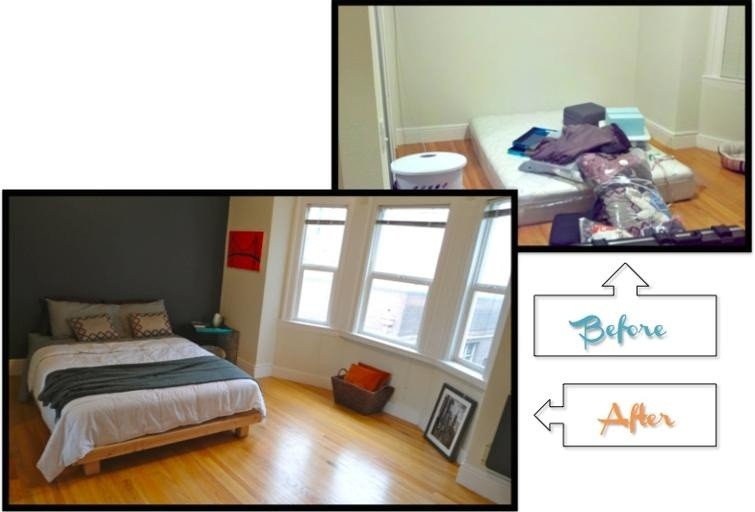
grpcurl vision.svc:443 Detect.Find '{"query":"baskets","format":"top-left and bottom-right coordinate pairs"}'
top-left (328, 368), bottom-right (395, 416)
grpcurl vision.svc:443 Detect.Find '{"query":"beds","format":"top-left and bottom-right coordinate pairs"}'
top-left (29, 332), bottom-right (260, 477)
top-left (468, 106), bottom-right (697, 226)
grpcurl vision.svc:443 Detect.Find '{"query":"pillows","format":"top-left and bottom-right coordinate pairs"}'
top-left (45, 297), bottom-right (173, 342)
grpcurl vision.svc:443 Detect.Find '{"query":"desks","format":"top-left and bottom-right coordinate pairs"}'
top-left (189, 325), bottom-right (239, 366)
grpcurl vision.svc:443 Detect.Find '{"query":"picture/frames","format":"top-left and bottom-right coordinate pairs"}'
top-left (422, 383), bottom-right (477, 462)
